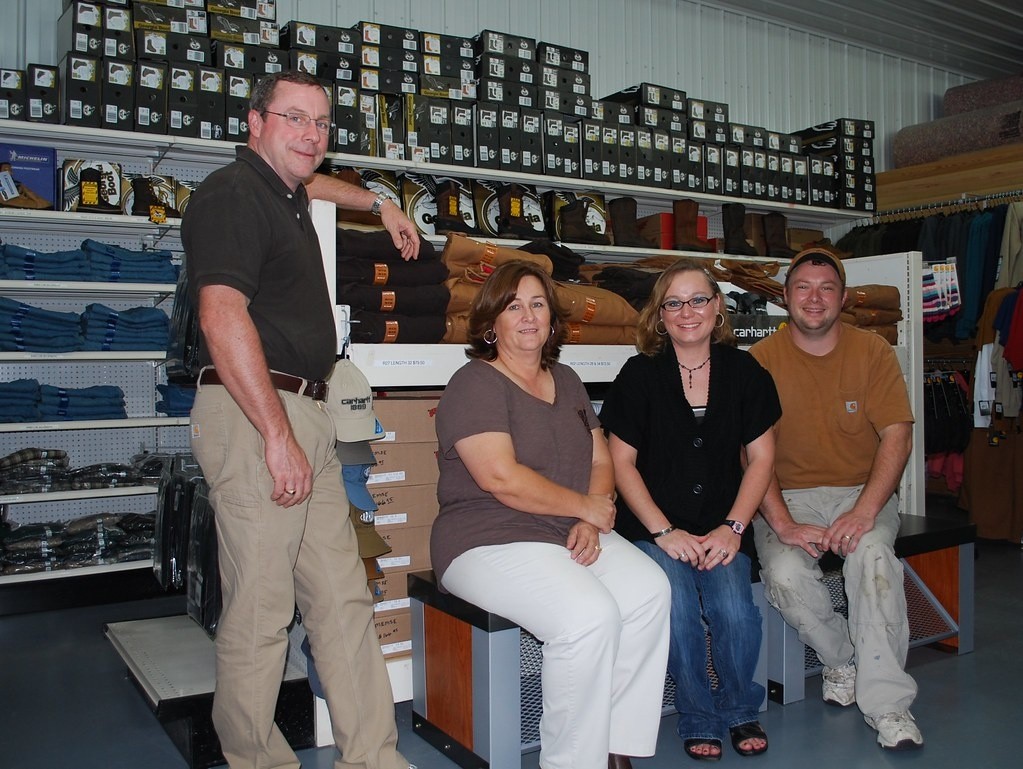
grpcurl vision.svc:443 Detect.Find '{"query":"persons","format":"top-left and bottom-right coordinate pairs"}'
top-left (181, 72), bottom-right (420, 769)
top-left (742, 248), bottom-right (923, 751)
top-left (597, 258), bottom-right (783, 761)
top-left (430, 260), bottom-right (673, 769)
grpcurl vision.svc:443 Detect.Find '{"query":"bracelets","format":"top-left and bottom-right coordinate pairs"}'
top-left (374, 191), bottom-right (390, 215)
top-left (651, 525), bottom-right (677, 539)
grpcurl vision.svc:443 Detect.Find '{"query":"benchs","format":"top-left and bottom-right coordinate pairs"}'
top-left (406, 568), bottom-right (768, 769)
top-left (767, 513), bottom-right (977, 706)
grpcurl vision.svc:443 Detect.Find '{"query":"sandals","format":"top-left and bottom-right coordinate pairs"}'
top-left (729, 721), bottom-right (768, 756)
top-left (685, 739), bottom-right (723, 761)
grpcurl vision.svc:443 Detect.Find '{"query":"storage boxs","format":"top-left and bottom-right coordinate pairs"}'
top-left (0, 0), bottom-right (877, 664)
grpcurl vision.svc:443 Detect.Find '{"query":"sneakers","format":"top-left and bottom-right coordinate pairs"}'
top-left (864, 710), bottom-right (924, 750)
top-left (822, 660), bottom-right (857, 706)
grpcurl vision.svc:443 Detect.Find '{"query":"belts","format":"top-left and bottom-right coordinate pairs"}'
top-left (200, 369), bottom-right (330, 402)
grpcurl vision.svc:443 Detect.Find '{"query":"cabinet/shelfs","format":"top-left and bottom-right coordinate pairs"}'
top-left (0, 118), bottom-right (923, 748)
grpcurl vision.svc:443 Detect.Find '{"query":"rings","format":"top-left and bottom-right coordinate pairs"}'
top-left (596, 547), bottom-right (603, 551)
top-left (842, 535), bottom-right (850, 539)
top-left (682, 554), bottom-right (686, 558)
top-left (721, 550), bottom-right (728, 556)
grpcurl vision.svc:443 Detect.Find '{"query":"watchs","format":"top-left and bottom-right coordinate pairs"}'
top-left (723, 519), bottom-right (745, 536)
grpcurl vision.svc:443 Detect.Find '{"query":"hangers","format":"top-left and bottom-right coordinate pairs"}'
top-left (838, 191), bottom-right (1023, 242)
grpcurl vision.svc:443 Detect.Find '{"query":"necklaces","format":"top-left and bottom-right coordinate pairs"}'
top-left (679, 356), bottom-right (710, 387)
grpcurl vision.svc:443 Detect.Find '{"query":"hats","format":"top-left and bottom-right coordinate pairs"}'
top-left (327, 359), bottom-right (391, 602)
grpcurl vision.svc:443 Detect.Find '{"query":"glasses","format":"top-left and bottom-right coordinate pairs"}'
top-left (660, 296), bottom-right (715, 312)
top-left (254, 107), bottom-right (336, 135)
